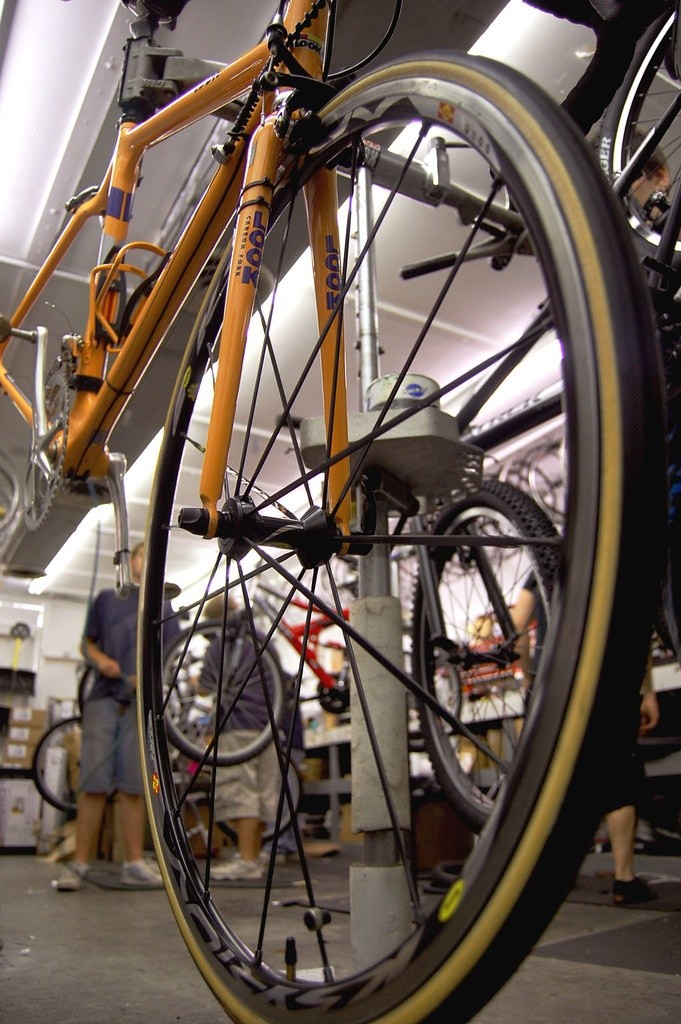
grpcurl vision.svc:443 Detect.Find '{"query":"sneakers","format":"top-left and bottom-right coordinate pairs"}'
top-left (120, 859), bottom-right (163, 888)
top-left (612, 877), bottom-right (657, 903)
top-left (259, 852), bottom-right (299, 864)
top-left (57, 861), bottom-right (90, 889)
top-left (209, 853), bottom-right (262, 881)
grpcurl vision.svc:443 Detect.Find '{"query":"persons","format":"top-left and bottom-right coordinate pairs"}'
top-left (501, 535), bottom-right (659, 904)
top-left (583, 123), bottom-right (681, 665)
top-left (257, 663), bottom-right (305, 868)
top-left (48, 540), bottom-right (171, 895)
top-left (186, 591), bottom-right (278, 883)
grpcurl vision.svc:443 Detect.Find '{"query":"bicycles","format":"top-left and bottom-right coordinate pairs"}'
top-left (0, 0), bottom-right (677, 1024)
